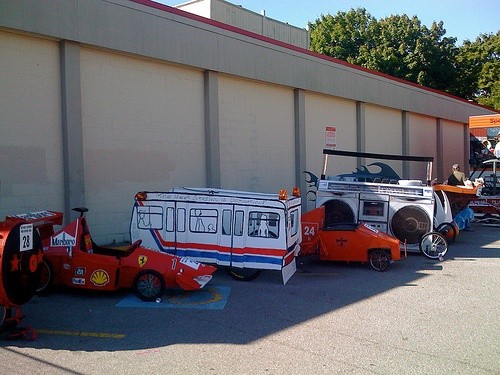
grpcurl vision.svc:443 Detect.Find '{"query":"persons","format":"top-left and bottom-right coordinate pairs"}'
top-left (448, 164), bottom-right (467, 185)
top-left (493, 132), bottom-right (500, 159)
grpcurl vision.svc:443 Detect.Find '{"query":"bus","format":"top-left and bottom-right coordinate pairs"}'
top-left (130, 187), bottom-right (303, 286)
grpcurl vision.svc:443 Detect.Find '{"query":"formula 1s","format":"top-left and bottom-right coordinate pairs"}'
top-left (297, 207), bottom-right (408, 271)
top-left (2, 208), bottom-right (218, 301)
